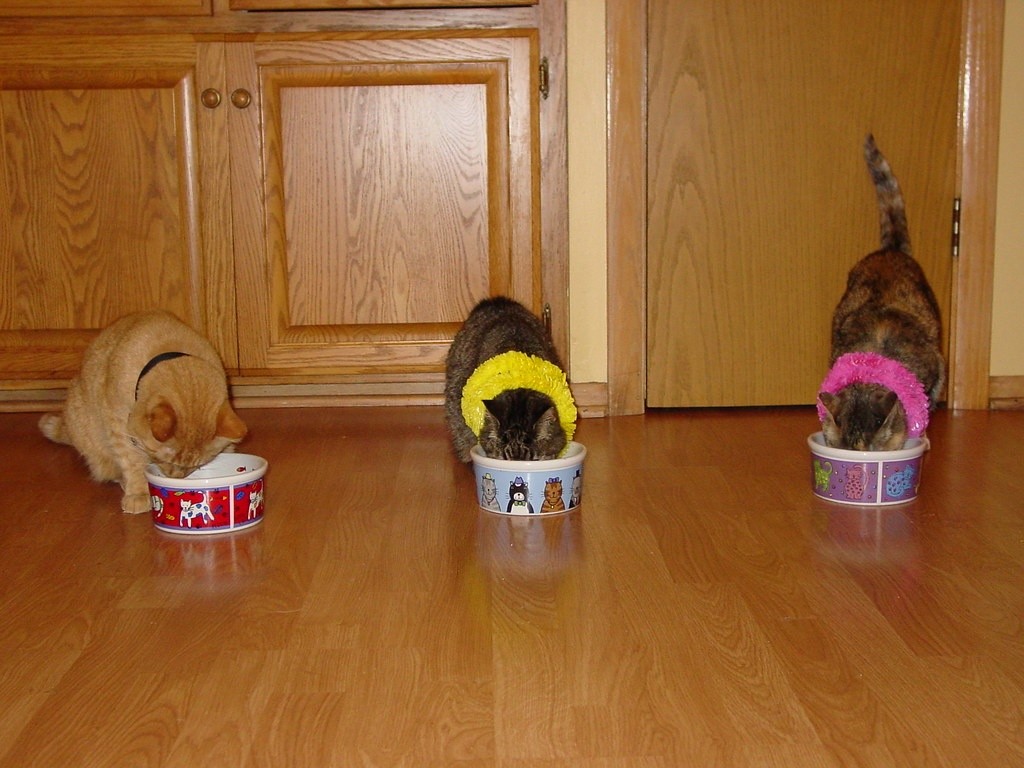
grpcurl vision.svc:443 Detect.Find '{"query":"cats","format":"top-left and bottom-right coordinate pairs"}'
top-left (441, 294), bottom-right (581, 465)
top-left (815, 131), bottom-right (947, 451)
top-left (37, 306), bottom-right (250, 515)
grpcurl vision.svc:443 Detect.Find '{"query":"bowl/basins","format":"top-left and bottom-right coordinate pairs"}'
top-left (470, 440), bottom-right (588, 521)
top-left (806, 430), bottom-right (927, 508)
top-left (144, 453), bottom-right (270, 537)
